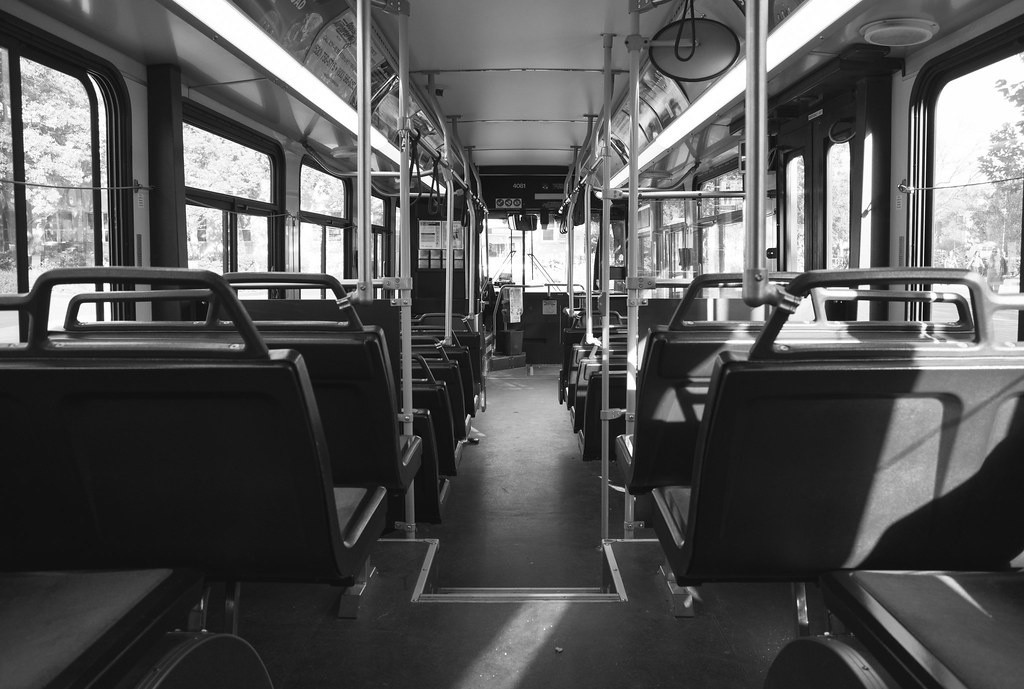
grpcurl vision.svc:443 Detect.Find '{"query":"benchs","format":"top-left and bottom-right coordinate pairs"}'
top-left (558, 265), bottom-right (1023, 689)
top-left (0, 266), bottom-right (487, 689)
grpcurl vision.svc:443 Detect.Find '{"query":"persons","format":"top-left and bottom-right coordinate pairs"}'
top-left (944, 247), bottom-right (1006, 295)
